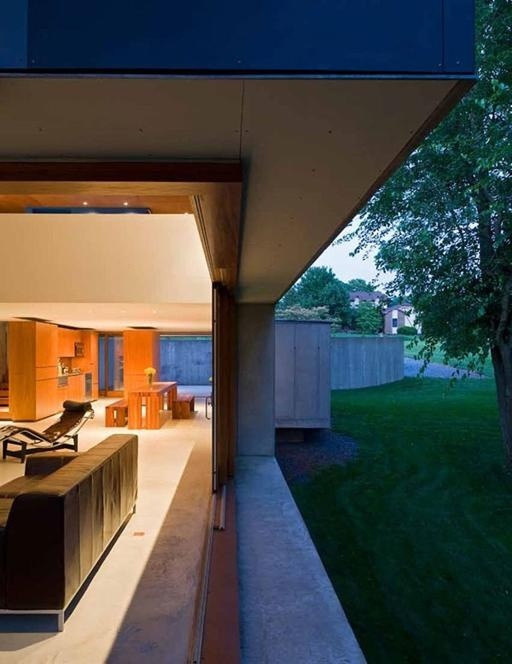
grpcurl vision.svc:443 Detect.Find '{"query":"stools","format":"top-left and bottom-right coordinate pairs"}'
top-left (172, 394), bottom-right (195, 418)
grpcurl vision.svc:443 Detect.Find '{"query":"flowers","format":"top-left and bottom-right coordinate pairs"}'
top-left (145, 367), bottom-right (157, 389)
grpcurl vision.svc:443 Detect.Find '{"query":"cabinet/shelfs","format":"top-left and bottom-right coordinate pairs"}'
top-left (67, 375), bottom-right (86, 402)
top-left (81, 331), bottom-right (99, 399)
top-left (7, 319), bottom-right (58, 421)
top-left (123, 330), bottom-right (159, 391)
top-left (58, 328), bottom-right (75, 358)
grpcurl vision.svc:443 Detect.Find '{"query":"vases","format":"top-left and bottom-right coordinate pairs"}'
top-left (147, 376), bottom-right (155, 391)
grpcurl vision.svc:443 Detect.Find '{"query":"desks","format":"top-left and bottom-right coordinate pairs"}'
top-left (127, 381), bottom-right (177, 429)
top-left (206, 395), bottom-right (212, 419)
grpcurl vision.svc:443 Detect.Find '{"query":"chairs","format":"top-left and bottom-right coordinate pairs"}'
top-left (0, 400), bottom-right (95, 463)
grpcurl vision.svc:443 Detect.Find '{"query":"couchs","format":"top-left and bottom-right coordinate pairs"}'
top-left (0, 433), bottom-right (139, 632)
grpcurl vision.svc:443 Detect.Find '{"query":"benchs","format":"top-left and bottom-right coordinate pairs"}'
top-left (105, 399), bottom-right (128, 428)
